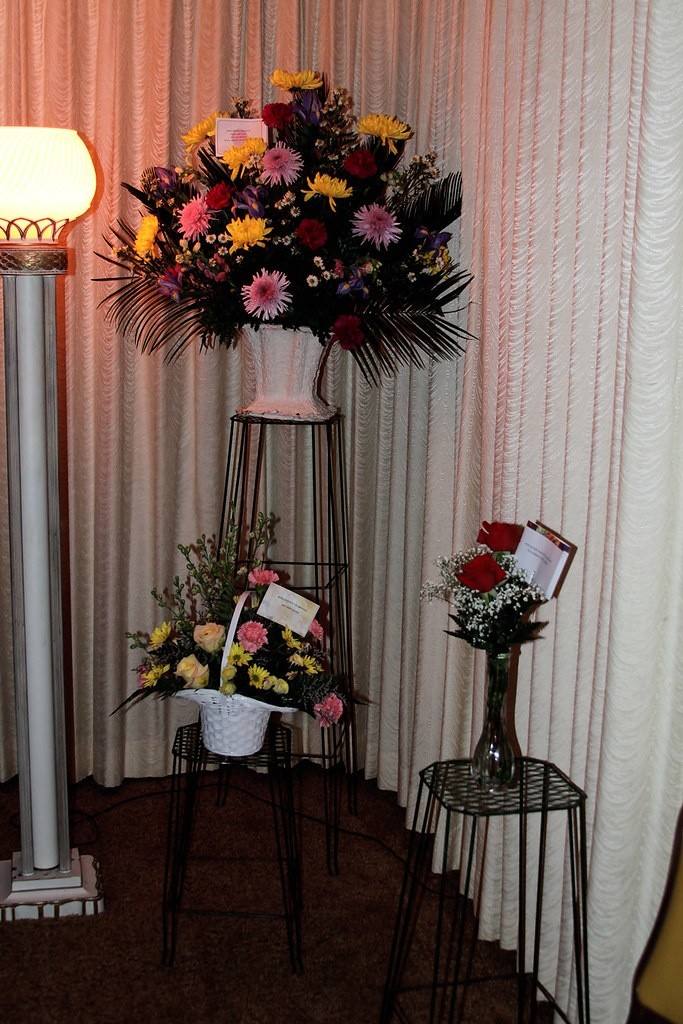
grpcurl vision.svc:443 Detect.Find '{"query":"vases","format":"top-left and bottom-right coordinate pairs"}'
top-left (235, 324), bottom-right (342, 422)
top-left (470, 649), bottom-right (524, 786)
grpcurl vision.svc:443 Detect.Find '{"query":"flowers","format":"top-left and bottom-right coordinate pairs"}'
top-left (417, 522), bottom-right (549, 784)
top-left (90, 69), bottom-right (482, 387)
top-left (108, 510), bottom-right (377, 728)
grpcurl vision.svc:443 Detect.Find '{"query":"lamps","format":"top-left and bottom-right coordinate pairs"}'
top-left (0, 125), bottom-right (105, 922)
